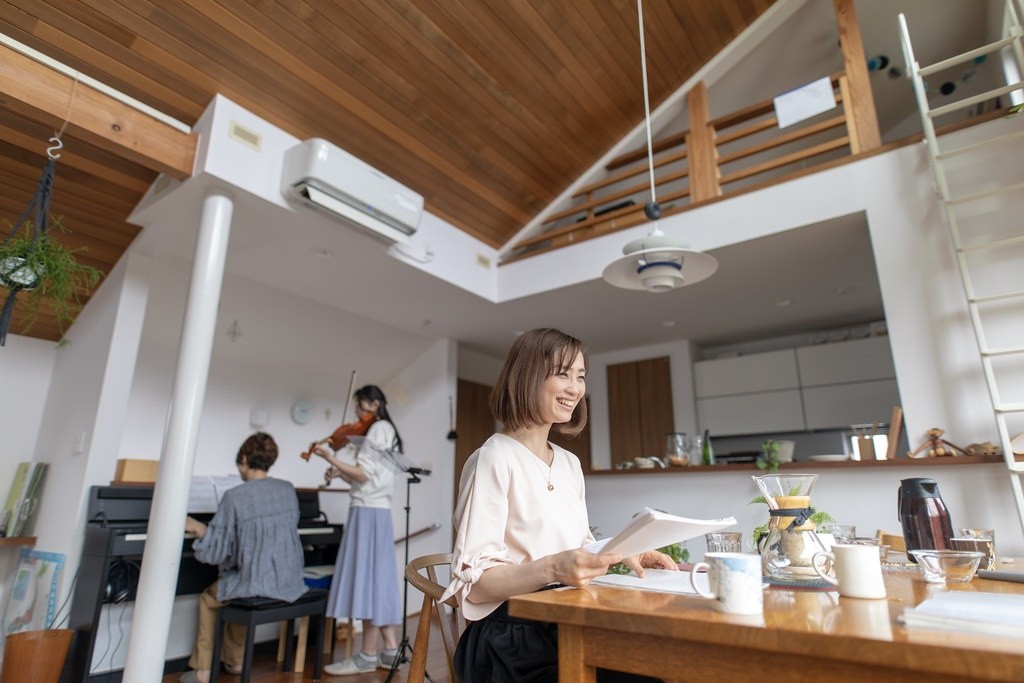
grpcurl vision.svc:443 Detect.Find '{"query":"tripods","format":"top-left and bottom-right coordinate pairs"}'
top-left (345, 435), bottom-right (435, 683)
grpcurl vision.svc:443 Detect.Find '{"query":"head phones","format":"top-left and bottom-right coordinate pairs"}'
top-left (102, 560), bottom-right (141, 605)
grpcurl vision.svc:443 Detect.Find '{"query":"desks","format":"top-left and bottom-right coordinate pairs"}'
top-left (508, 558), bottom-right (1024, 683)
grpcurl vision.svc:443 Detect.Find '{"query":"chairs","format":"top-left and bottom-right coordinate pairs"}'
top-left (875, 530), bottom-right (907, 553)
top-left (405, 554), bottom-right (459, 683)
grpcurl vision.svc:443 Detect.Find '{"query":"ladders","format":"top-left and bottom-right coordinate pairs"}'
top-left (898, 13), bottom-right (1024, 529)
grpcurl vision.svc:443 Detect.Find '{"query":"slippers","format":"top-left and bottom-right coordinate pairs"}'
top-left (180, 670), bottom-right (201, 683)
top-left (323, 657), bottom-right (376, 675)
top-left (219, 660), bottom-right (242, 675)
top-left (376, 652), bottom-right (401, 670)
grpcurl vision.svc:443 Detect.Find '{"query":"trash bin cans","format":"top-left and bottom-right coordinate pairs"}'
top-left (0, 630), bottom-right (74, 683)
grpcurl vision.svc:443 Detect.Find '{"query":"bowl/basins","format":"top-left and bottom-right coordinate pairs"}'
top-left (634, 456), bottom-right (655, 468)
top-left (908, 548), bottom-right (986, 582)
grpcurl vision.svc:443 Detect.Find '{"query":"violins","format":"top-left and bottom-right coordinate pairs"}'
top-left (299, 410), bottom-right (382, 463)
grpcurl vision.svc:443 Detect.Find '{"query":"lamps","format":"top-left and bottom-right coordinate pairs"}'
top-left (602, 0), bottom-right (718, 293)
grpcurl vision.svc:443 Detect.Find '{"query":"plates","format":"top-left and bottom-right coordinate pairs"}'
top-left (807, 454), bottom-right (849, 461)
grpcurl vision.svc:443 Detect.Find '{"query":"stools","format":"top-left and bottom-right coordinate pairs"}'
top-left (209, 590), bottom-right (330, 683)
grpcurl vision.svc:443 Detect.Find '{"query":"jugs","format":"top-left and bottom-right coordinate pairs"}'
top-left (898, 477), bottom-right (956, 563)
top-left (751, 473), bottom-right (831, 580)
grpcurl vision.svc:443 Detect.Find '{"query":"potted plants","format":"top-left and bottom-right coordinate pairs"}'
top-left (0, 214), bottom-right (106, 350)
top-left (747, 441), bottom-right (837, 579)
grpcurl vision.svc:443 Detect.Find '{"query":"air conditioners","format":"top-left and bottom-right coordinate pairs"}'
top-left (281, 137), bottom-right (425, 247)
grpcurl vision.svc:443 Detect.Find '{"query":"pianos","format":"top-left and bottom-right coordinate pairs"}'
top-left (63, 480), bottom-right (344, 683)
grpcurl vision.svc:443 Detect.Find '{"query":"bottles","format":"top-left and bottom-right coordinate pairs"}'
top-left (664, 429), bottom-right (718, 465)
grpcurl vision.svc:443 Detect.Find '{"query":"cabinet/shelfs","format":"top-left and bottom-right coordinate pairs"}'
top-left (693, 335), bottom-right (901, 438)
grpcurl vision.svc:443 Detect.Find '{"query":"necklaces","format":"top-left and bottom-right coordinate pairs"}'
top-left (516, 432), bottom-right (554, 491)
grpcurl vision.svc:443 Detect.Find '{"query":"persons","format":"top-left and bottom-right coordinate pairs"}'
top-left (437, 327), bottom-right (678, 683)
top-left (180, 433), bottom-right (310, 683)
top-left (313, 385), bottom-right (403, 674)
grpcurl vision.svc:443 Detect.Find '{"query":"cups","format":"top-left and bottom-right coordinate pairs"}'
top-left (831, 526), bottom-right (890, 563)
top-left (812, 544), bottom-right (886, 599)
top-left (777, 440), bottom-right (796, 464)
top-left (950, 528), bottom-right (996, 571)
top-left (691, 553), bottom-right (763, 614)
top-left (706, 533), bottom-right (742, 552)
